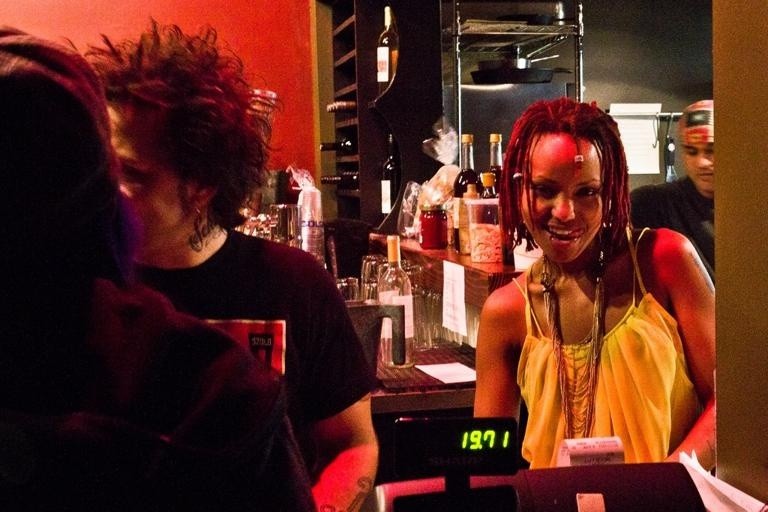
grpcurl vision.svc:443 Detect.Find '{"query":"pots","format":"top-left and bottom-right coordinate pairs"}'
top-left (495, 12), bottom-right (575, 25)
top-left (472, 54), bottom-right (575, 83)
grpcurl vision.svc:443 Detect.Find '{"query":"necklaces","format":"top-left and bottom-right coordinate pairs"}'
top-left (538, 236), bottom-right (607, 440)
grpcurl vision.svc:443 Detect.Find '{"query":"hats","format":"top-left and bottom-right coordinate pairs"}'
top-left (674, 100), bottom-right (714, 145)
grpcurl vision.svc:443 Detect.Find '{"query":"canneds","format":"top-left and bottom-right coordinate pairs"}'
top-left (419, 204), bottom-right (448, 249)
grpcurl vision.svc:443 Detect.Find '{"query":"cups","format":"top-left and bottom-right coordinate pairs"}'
top-left (299, 188), bottom-right (324, 263)
top-left (466, 198), bottom-right (502, 264)
top-left (335, 277), bottom-right (361, 301)
top-left (357, 254), bottom-right (466, 354)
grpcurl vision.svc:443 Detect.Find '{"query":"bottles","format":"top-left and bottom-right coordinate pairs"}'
top-left (312, 95), bottom-right (360, 189)
top-left (378, 233), bottom-right (417, 371)
top-left (452, 134), bottom-right (504, 256)
top-left (552, 1), bottom-right (566, 26)
top-left (379, 134), bottom-right (398, 226)
top-left (377, 5), bottom-right (400, 93)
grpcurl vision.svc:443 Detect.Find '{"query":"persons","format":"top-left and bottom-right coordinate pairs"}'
top-left (627, 98), bottom-right (714, 287)
top-left (0, 22), bottom-right (319, 510)
top-left (77, 15), bottom-right (380, 512)
top-left (472, 95), bottom-right (715, 478)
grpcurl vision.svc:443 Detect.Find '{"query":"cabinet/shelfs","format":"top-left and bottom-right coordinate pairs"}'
top-left (330, 0), bottom-right (443, 276)
